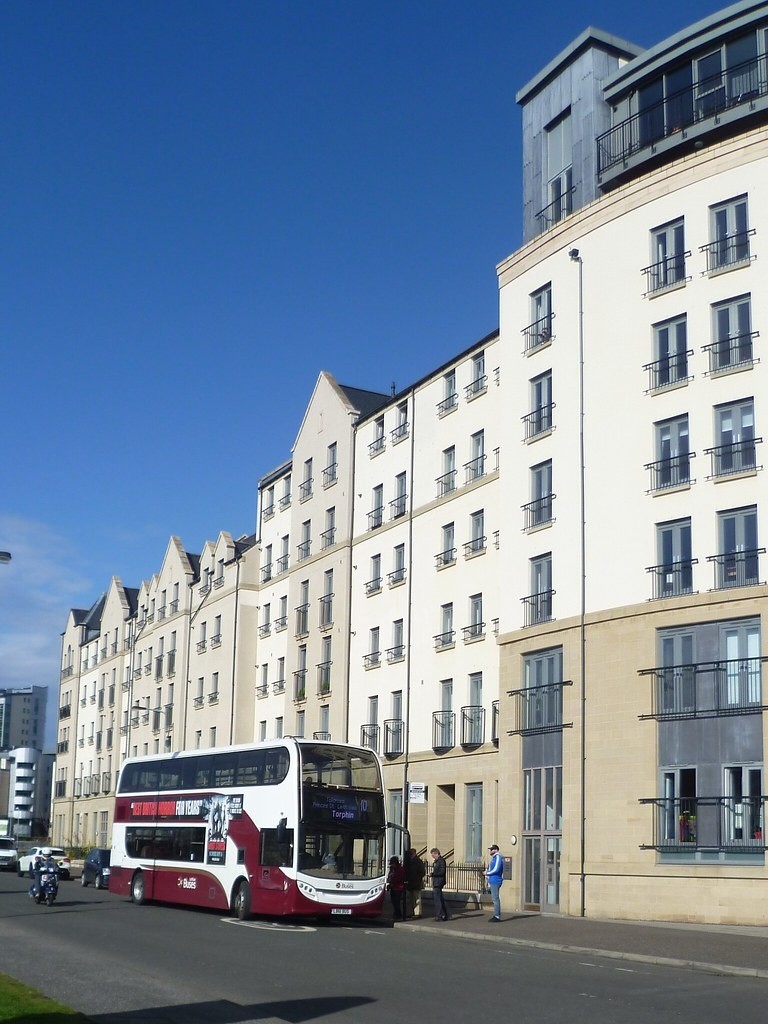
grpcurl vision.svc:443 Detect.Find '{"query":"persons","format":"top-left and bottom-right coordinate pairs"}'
top-left (405, 848), bottom-right (425, 920)
top-left (387, 857), bottom-right (406, 920)
top-left (428, 847), bottom-right (447, 922)
top-left (34, 848), bottom-right (59, 902)
top-left (482, 844), bottom-right (503, 922)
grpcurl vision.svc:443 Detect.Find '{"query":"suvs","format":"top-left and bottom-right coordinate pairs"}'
top-left (16, 847), bottom-right (70, 879)
top-left (81, 849), bottom-right (111, 889)
top-left (0, 836), bottom-right (18, 872)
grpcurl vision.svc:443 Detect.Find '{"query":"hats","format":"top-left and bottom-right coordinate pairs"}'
top-left (389, 856), bottom-right (398, 865)
top-left (487, 845), bottom-right (499, 850)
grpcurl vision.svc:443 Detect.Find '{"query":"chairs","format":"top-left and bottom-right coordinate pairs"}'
top-left (129, 838), bottom-right (200, 861)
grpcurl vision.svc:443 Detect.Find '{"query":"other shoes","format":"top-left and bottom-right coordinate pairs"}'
top-left (391, 917), bottom-right (403, 922)
top-left (431, 915), bottom-right (447, 922)
top-left (407, 914), bottom-right (420, 919)
top-left (488, 916), bottom-right (501, 923)
top-left (35, 892), bottom-right (40, 898)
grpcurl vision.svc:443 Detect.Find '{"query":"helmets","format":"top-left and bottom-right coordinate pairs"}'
top-left (40, 848), bottom-right (52, 860)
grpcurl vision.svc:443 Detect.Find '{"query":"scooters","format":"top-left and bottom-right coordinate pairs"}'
top-left (29, 862), bottom-right (63, 906)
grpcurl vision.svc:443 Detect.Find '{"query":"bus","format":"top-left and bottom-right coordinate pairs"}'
top-left (108, 735), bottom-right (411, 919)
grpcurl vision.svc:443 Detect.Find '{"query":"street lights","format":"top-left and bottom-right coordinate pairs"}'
top-left (16, 807), bottom-right (20, 846)
